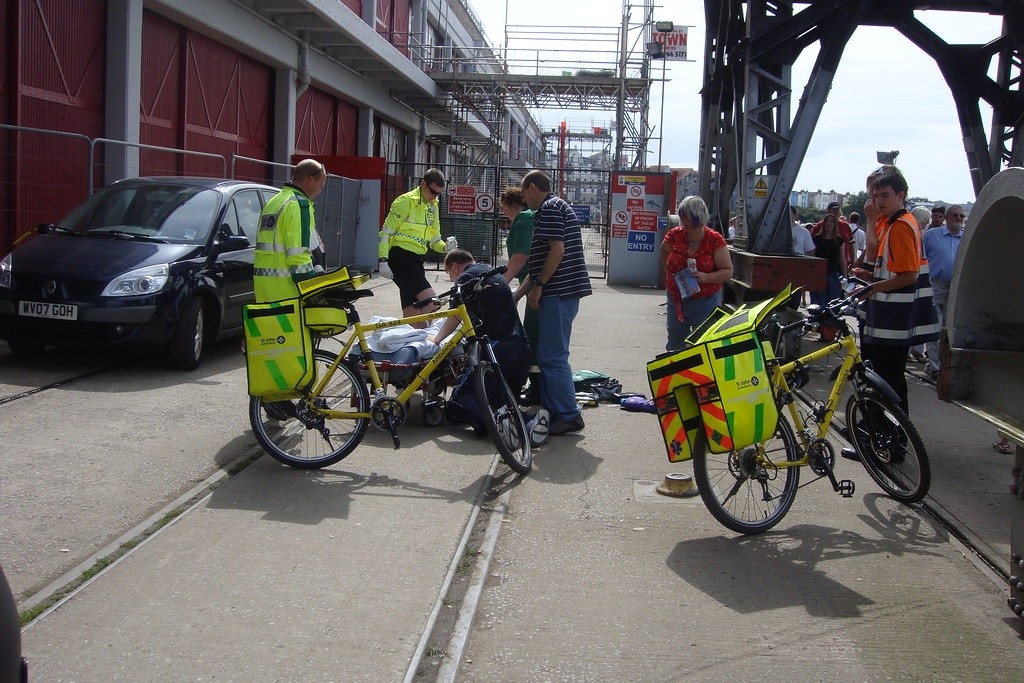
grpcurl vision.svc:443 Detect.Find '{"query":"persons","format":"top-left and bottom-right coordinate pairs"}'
top-left (426, 249), bottom-right (552, 450)
top-left (508, 170), bottom-right (594, 436)
top-left (499, 187), bottom-right (540, 377)
top-left (659, 196), bottom-right (734, 350)
top-left (378, 168), bottom-right (456, 329)
top-left (252, 158), bottom-right (328, 418)
top-left (729, 164), bottom-right (966, 463)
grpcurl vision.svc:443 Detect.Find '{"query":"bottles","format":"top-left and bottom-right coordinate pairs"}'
top-left (839, 276), bottom-right (865, 305)
top-left (687, 256), bottom-right (697, 272)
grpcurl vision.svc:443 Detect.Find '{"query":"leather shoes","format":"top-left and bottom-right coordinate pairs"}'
top-left (841, 444), bottom-right (905, 464)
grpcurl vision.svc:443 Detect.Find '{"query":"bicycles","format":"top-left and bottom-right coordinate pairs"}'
top-left (691, 283), bottom-right (932, 535)
top-left (248, 263), bottom-right (533, 475)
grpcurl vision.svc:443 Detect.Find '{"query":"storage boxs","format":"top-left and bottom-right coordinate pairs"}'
top-left (600, 129), bottom-right (607, 134)
top-left (552, 129), bottom-right (555, 132)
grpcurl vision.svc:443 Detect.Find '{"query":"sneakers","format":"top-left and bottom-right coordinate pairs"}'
top-left (548, 416), bottom-right (585, 435)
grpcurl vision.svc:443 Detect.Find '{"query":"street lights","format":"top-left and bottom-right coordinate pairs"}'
top-left (646, 21), bottom-right (673, 172)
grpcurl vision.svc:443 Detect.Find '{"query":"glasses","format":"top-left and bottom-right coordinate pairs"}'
top-left (946, 213), bottom-right (966, 219)
top-left (423, 179), bottom-right (443, 196)
top-left (308, 164), bottom-right (325, 179)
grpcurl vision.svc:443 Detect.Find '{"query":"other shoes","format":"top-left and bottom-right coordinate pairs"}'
top-left (912, 350), bottom-right (928, 363)
top-left (520, 385), bottom-right (541, 405)
top-left (800, 301), bottom-right (805, 308)
top-left (924, 362), bottom-right (940, 380)
top-left (445, 407), bottom-right (463, 423)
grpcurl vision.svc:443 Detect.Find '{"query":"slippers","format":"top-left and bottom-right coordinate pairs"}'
top-left (992, 439), bottom-right (1012, 454)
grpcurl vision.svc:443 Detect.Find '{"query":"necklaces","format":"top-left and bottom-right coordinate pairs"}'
top-left (690, 239), bottom-right (696, 246)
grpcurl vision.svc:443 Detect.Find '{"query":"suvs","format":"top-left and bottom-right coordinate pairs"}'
top-left (0, 175), bottom-right (284, 372)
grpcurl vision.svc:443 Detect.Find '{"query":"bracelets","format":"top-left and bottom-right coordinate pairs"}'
top-left (534, 278), bottom-right (545, 287)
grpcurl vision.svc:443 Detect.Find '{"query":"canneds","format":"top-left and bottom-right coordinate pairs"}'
top-left (687, 257), bottom-right (698, 274)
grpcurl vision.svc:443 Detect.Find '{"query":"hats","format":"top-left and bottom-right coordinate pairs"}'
top-left (827, 201), bottom-right (840, 211)
